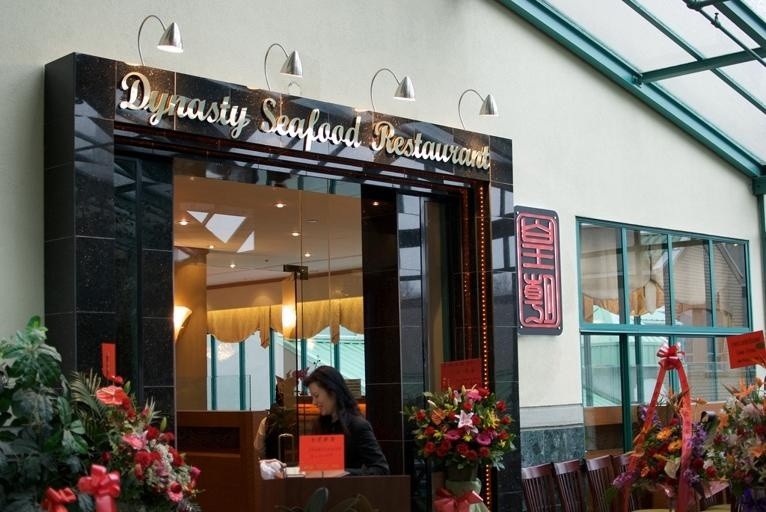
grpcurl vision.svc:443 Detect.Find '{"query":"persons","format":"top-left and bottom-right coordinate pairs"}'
top-left (253, 383), bottom-right (284, 458)
top-left (301, 365), bottom-right (391, 475)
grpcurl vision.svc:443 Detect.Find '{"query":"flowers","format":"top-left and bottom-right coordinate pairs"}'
top-left (398, 382), bottom-right (520, 512)
top-left (601, 376), bottom-right (766, 511)
top-left (67, 367), bottom-right (208, 512)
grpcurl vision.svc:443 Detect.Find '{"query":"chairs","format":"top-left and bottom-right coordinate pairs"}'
top-left (521, 450), bottom-right (638, 512)
